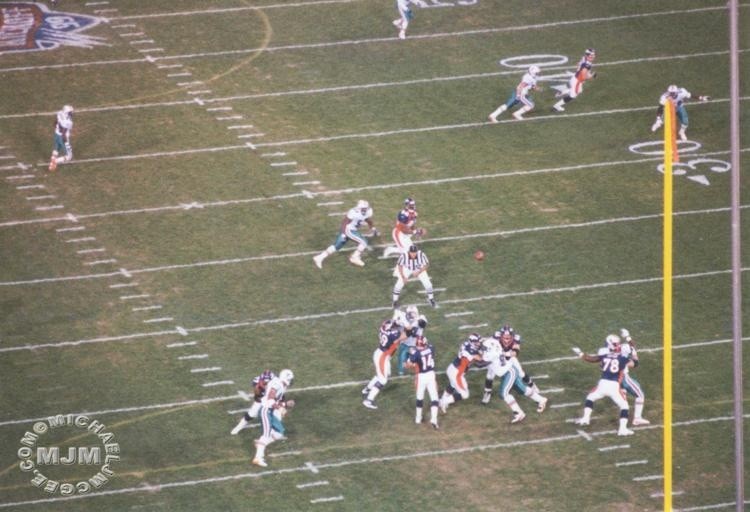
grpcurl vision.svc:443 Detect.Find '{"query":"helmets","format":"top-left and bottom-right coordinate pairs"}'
top-left (357, 200), bottom-right (370, 208)
top-left (606, 334), bottom-right (622, 352)
top-left (468, 325), bottom-right (514, 361)
top-left (393, 305), bottom-right (419, 326)
top-left (415, 335), bottom-right (428, 351)
top-left (404, 197), bottom-right (416, 213)
top-left (260, 369), bottom-right (274, 382)
top-left (528, 49), bottom-right (678, 98)
top-left (62, 104), bottom-right (74, 118)
top-left (279, 369), bottom-right (294, 387)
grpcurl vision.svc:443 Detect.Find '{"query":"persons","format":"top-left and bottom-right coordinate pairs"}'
top-left (392, 0), bottom-right (414, 39)
top-left (652, 85), bottom-right (711, 140)
top-left (489, 65), bottom-right (543, 123)
top-left (553, 49), bottom-right (596, 112)
top-left (48, 105), bottom-right (74, 170)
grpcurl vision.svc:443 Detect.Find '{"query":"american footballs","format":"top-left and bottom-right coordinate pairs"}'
top-left (474, 251), bottom-right (485, 261)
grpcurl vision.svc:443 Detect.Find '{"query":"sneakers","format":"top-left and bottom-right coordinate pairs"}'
top-left (632, 417), bottom-right (651, 425)
top-left (531, 383), bottom-right (548, 414)
top-left (48, 154), bottom-right (58, 171)
top-left (488, 103), bottom-right (564, 123)
top-left (616, 429), bottom-right (635, 437)
top-left (416, 398), bottom-right (449, 428)
top-left (361, 386), bottom-right (378, 410)
top-left (230, 421), bottom-right (268, 467)
top-left (312, 247), bottom-right (439, 307)
top-left (481, 387), bottom-right (493, 405)
top-left (510, 411), bottom-right (527, 424)
top-left (575, 418), bottom-right (590, 426)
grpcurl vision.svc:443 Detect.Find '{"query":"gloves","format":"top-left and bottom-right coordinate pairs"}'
top-left (620, 327), bottom-right (630, 337)
top-left (65, 141), bottom-right (73, 153)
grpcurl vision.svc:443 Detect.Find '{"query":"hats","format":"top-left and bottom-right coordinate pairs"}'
top-left (408, 245), bottom-right (418, 253)
